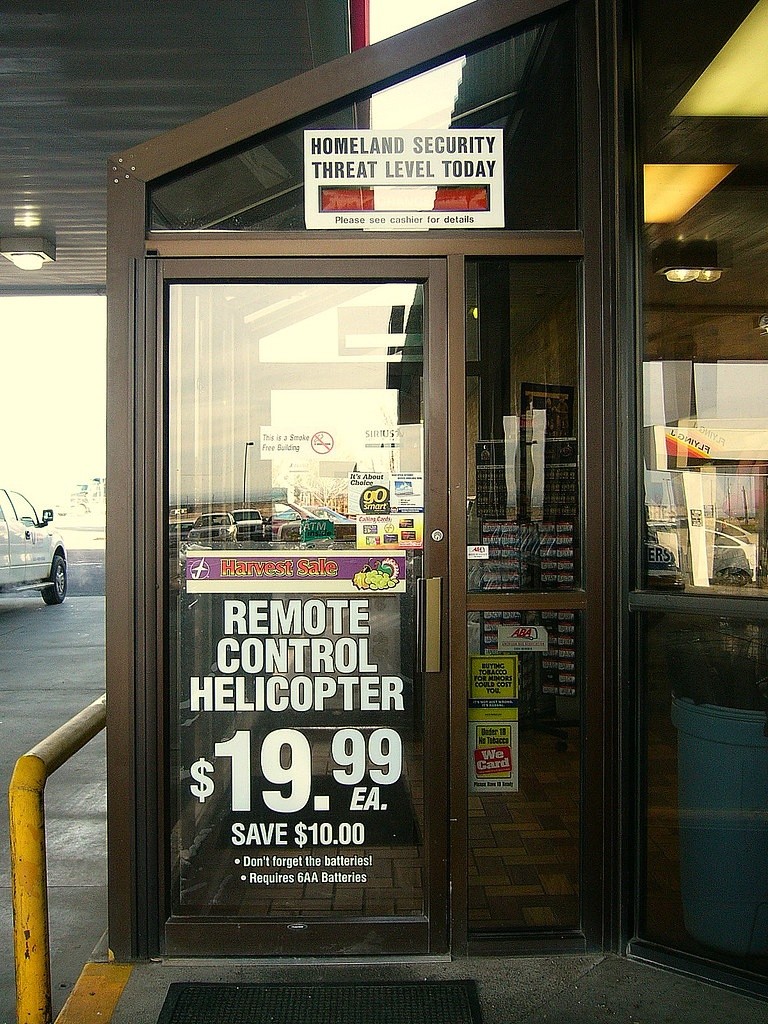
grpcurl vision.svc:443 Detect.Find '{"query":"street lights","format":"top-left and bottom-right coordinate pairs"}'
top-left (243, 442), bottom-right (254, 508)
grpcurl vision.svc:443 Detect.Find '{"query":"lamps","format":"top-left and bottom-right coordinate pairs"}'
top-left (0, 238), bottom-right (56, 272)
top-left (652, 240), bottom-right (734, 284)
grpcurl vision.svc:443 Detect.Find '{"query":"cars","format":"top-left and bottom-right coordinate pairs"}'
top-left (169, 502), bottom-right (356, 548)
top-left (646, 515), bottom-right (759, 586)
top-left (0, 487), bottom-right (69, 605)
